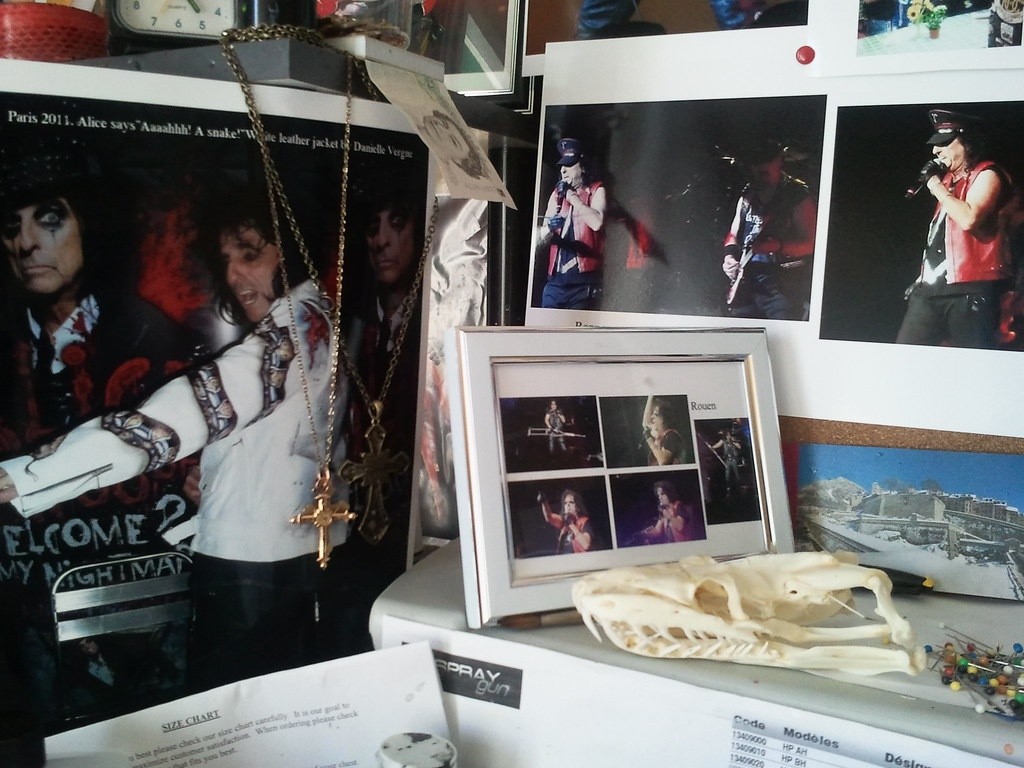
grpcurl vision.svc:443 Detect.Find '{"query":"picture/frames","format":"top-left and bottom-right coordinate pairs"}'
top-left (446, 322), bottom-right (797, 627)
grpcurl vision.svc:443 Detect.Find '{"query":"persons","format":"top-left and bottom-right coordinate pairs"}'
top-left (722, 142), bottom-right (819, 321)
top-left (417, 109), bottom-right (482, 180)
top-left (608, 163), bottom-right (689, 312)
top-left (78, 636), bottom-right (127, 706)
top-left (1, 156), bottom-right (194, 460)
top-left (895, 109), bottom-right (1013, 351)
top-left (1, 166), bottom-right (351, 694)
top-left (537, 396), bottom-right (745, 555)
top-left (540, 137), bottom-right (608, 312)
top-left (349, 162), bottom-right (428, 522)
top-left (574, 0), bottom-right (769, 42)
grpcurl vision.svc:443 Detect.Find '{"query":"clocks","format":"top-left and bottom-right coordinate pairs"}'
top-left (107, 0), bottom-right (243, 44)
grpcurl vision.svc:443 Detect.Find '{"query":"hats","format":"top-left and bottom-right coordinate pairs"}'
top-left (926, 109), bottom-right (981, 144)
top-left (556, 138), bottom-right (587, 165)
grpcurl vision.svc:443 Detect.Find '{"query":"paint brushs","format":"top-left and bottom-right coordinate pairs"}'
top-left (496, 609), bottom-right (597, 629)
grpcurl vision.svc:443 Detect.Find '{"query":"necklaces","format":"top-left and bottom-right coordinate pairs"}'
top-left (218, 24), bottom-right (442, 575)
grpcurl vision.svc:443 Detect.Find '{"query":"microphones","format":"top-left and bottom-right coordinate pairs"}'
top-left (638, 426), bottom-right (652, 449)
top-left (658, 510), bottom-right (662, 520)
top-left (904, 158), bottom-right (942, 199)
top-left (557, 180), bottom-right (567, 213)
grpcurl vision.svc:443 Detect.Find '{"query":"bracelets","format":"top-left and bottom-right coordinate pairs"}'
top-left (779, 239), bottom-right (784, 253)
top-left (569, 192), bottom-right (578, 202)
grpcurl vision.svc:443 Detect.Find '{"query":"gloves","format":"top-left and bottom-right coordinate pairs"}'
top-left (548, 214), bottom-right (565, 232)
top-left (921, 160), bottom-right (944, 194)
top-left (556, 181), bottom-right (574, 200)
